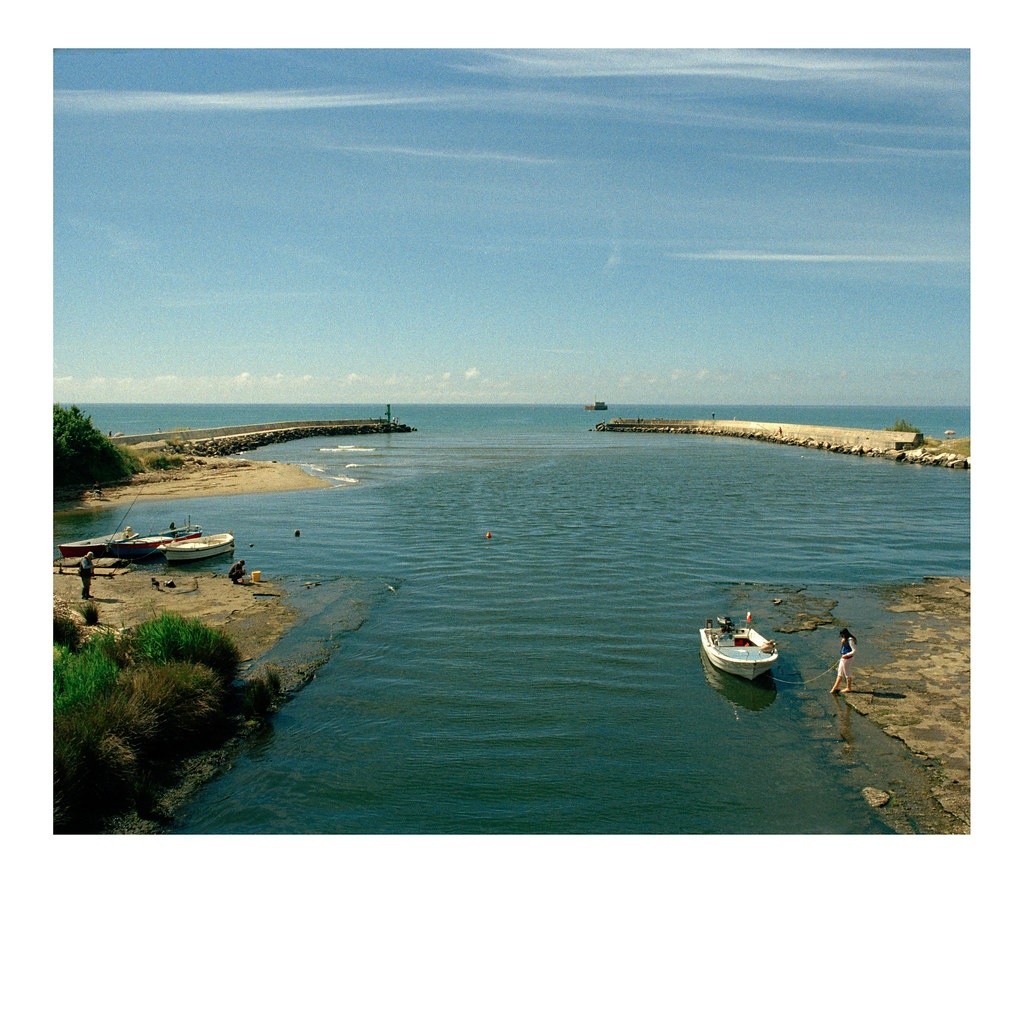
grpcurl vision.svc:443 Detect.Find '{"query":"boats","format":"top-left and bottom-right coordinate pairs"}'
top-left (700, 619), bottom-right (779, 680)
top-left (584, 402), bottom-right (608, 411)
top-left (107, 524), bottom-right (202, 562)
top-left (699, 646), bottom-right (778, 713)
top-left (58, 530), bottom-right (140, 558)
top-left (157, 532), bottom-right (234, 561)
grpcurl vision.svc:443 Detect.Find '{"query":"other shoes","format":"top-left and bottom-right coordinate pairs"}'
top-left (82, 596), bottom-right (88, 600)
top-left (829, 686), bottom-right (838, 693)
top-left (88, 594), bottom-right (93, 598)
top-left (231, 578), bottom-right (237, 581)
top-left (841, 688), bottom-right (851, 693)
top-left (233, 581), bottom-right (238, 584)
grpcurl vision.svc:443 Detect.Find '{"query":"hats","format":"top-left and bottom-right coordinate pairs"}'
top-left (88, 552), bottom-right (95, 559)
top-left (839, 628), bottom-right (848, 637)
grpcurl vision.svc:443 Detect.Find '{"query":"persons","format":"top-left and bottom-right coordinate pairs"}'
top-left (77, 551), bottom-right (94, 600)
top-left (829, 628), bottom-right (858, 693)
top-left (393, 416), bottom-right (399, 425)
top-left (228, 560), bottom-right (246, 584)
top-left (108, 431), bottom-right (112, 437)
